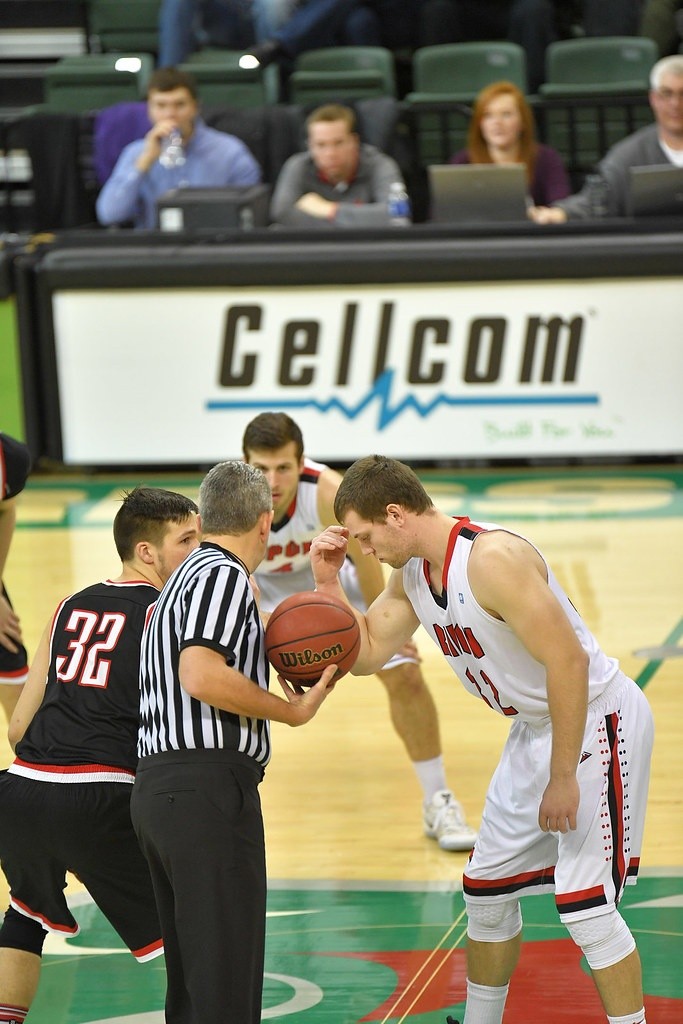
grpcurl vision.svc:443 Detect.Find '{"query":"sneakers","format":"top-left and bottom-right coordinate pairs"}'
top-left (424, 790), bottom-right (478, 852)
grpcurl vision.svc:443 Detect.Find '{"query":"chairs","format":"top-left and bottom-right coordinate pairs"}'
top-left (43, 0), bottom-right (659, 161)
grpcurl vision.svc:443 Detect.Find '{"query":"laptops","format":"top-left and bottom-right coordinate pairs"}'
top-left (628, 163), bottom-right (683, 218)
top-left (427, 164), bottom-right (530, 220)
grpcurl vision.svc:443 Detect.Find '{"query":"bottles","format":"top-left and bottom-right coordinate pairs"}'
top-left (158, 128), bottom-right (187, 170)
top-left (385, 181), bottom-right (413, 227)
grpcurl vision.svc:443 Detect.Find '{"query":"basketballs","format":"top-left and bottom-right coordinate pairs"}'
top-left (264, 591), bottom-right (363, 687)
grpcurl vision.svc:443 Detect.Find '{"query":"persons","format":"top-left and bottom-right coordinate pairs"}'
top-left (94, 69), bottom-right (262, 229)
top-left (1, 487), bottom-right (201, 1024)
top-left (226, 413), bottom-right (479, 852)
top-left (133, 459), bottom-right (343, 1023)
top-left (448, 82), bottom-right (573, 210)
top-left (307, 455), bottom-right (654, 1024)
top-left (269, 105), bottom-right (411, 228)
top-left (527, 54), bottom-right (683, 226)
top-left (0, 432), bottom-right (30, 722)
